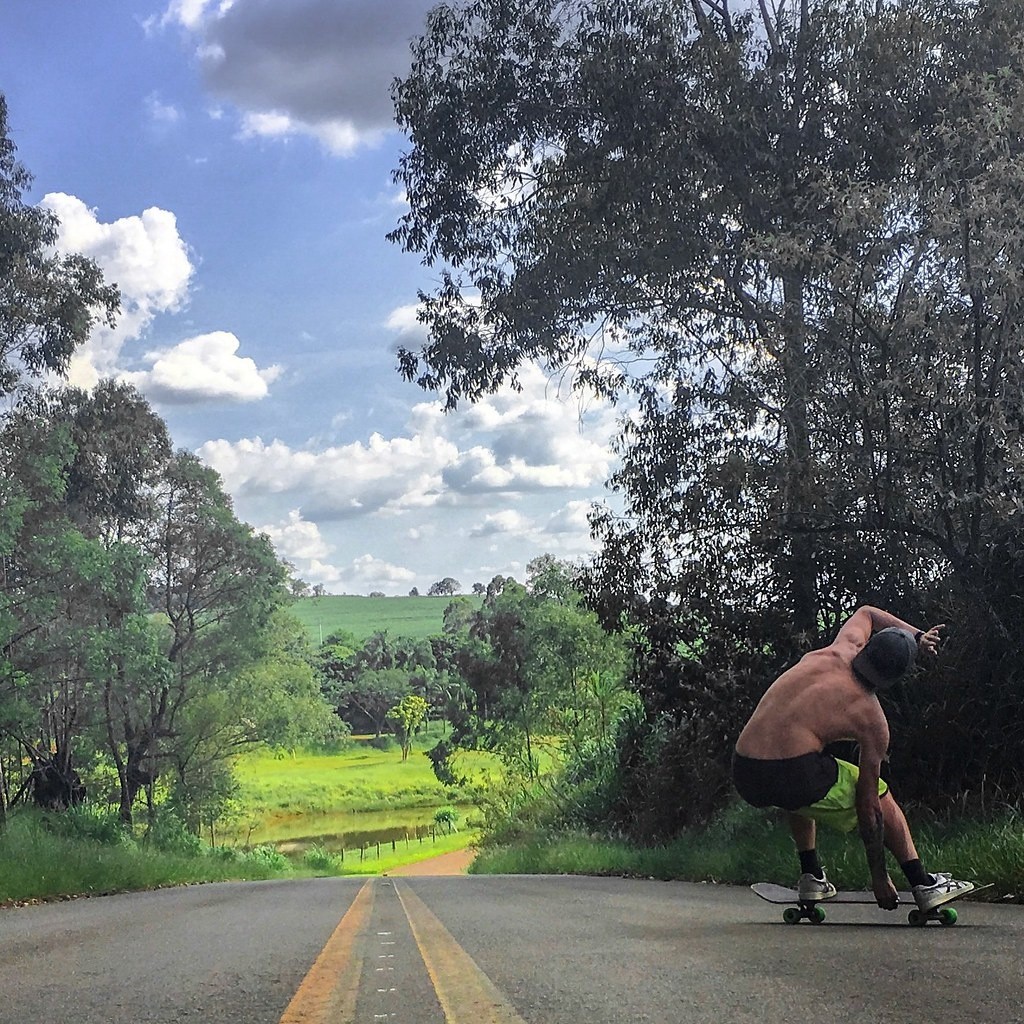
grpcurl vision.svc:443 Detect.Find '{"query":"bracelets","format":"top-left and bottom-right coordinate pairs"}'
top-left (914, 631), bottom-right (925, 642)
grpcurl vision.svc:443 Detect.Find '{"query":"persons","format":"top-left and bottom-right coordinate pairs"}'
top-left (730, 605), bottom-right (971, 911)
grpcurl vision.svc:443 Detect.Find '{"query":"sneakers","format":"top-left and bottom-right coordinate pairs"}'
top-left (797, 872), bottom-right (839, 901)
top-left (912, 873), bottom-right (975, 910)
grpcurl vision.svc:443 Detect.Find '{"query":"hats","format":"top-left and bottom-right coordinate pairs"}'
top-left (853, 626), bottom-right (916, 691)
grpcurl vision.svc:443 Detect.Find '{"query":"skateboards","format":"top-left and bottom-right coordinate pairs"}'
top-left (750, 876), bottom-right (996, 926)
